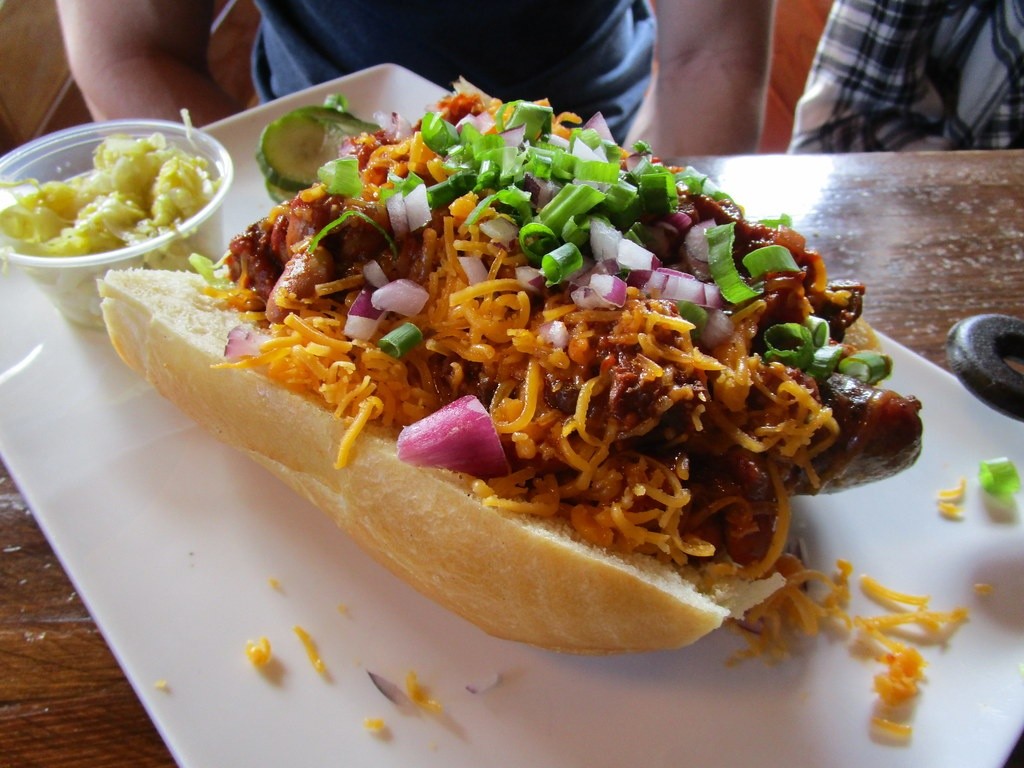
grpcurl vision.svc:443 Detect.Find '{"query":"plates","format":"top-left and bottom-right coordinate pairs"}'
top-left (0, 62), bottom-right (1024, 768)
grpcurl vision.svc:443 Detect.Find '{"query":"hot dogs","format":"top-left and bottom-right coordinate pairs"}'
top-left (97, 84), bottom-right (927, 653)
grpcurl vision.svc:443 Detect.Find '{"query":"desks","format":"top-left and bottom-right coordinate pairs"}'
top-left (1, 149), bottom-right (1024, 768)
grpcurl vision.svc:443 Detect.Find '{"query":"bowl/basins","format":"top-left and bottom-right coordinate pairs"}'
top-left (0, 119), bottom-right (233, 329)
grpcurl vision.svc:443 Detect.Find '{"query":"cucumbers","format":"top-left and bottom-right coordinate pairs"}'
top-left (255, 106), bottom-right (383, 193)
top-left (264, 178), bottom-right (299, 203)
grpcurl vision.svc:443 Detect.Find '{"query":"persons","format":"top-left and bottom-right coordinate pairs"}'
top-left (57, 1), bottom-right (775, 156)
top-left (784, 0), bottom-right (1024, 153)
